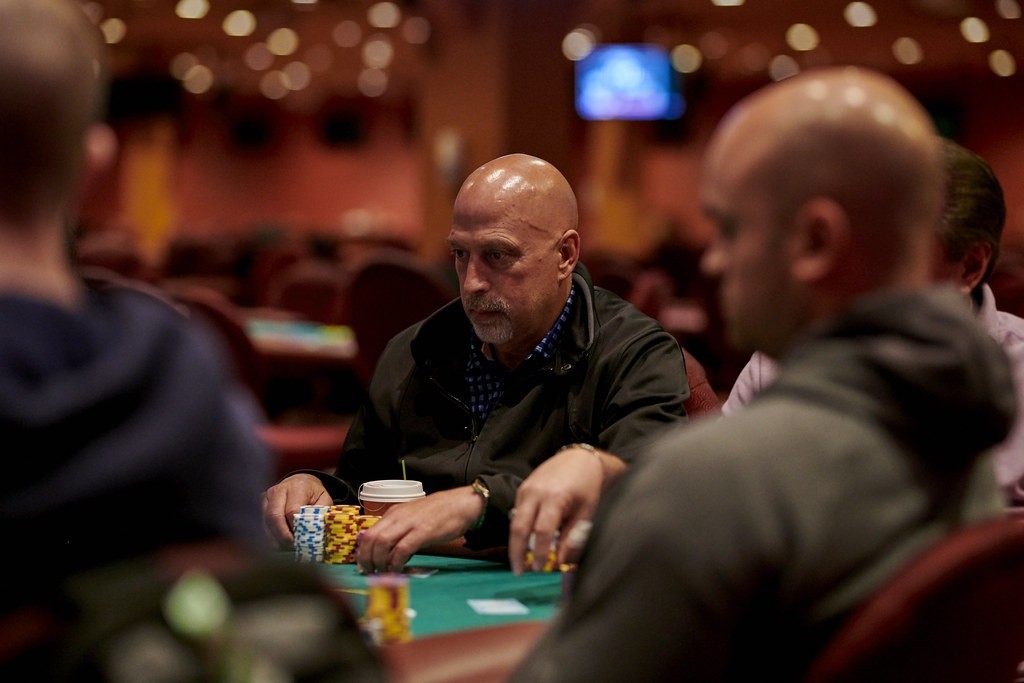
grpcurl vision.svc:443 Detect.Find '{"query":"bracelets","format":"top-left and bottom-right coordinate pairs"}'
top-left (555, 443), bottom-right (602, 459)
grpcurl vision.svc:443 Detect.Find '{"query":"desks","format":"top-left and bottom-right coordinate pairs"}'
top-left (285, 534), bottom-right (577, 655)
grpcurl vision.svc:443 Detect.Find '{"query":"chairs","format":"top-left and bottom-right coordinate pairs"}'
top-left (798, 505), bottom-right (1024, 683)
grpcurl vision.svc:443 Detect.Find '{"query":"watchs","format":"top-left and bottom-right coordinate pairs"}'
top-left (472, 477), bottom-right (489, 527)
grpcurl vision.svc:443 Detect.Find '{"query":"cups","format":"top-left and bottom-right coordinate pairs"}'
top-left (358, 480), bottom-right (426, 516)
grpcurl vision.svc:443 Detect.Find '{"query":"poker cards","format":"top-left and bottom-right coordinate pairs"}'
top-left (467, 597), bottom-right (530, 617)
top-left (401, 565), bottom-right (440, 579)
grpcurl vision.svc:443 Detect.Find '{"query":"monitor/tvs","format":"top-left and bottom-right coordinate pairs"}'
top-left (571, 43), bottom-right (687, 121)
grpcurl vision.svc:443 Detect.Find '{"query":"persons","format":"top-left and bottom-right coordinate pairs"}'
top-left (508, 65), bottom-right (1017, 683)
top-left (0, 0), bottom-right (270, 562)
top-left (264, 152), bottom-right (691, 574)
top-left (509, 136), bottom-right (1024, 576)
